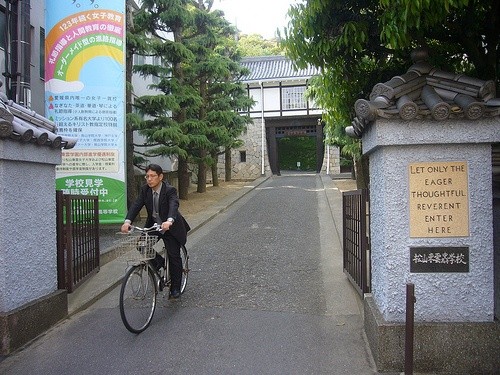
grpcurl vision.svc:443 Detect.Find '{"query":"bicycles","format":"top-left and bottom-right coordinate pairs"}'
top-left (114, 223), bottom-right (202, 335)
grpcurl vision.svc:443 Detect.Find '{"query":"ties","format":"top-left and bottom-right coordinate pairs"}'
top-left (154, 192), bottom-right (161, 225)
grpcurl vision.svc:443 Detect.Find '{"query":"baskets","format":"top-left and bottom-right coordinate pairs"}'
top-left (112, 236), bottom-right (158, 262)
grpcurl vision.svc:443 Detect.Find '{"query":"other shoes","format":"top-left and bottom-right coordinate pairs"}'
top-left (169, 290), bottom-right (181, 300)
top-left (149, 259), bottom-right (165, 274)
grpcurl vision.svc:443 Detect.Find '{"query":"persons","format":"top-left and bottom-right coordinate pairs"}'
top-left (121, 164), bottom-right (192, 301)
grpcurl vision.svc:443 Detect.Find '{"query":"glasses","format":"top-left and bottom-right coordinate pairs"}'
top-left (144, 174), bottom-right (159, 180)
